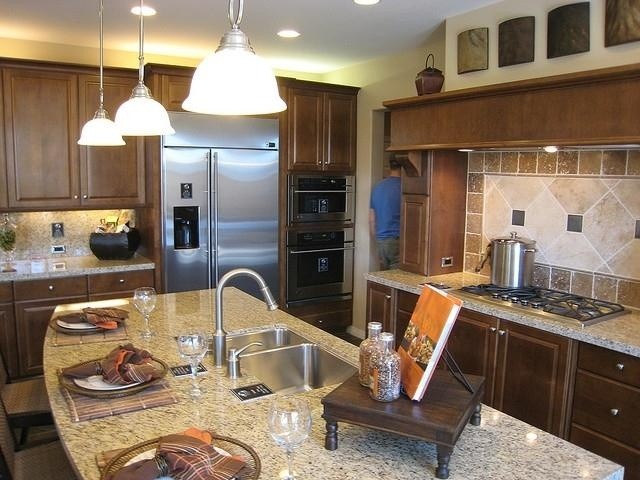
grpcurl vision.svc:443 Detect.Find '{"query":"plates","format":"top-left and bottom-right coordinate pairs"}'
top-left (73, 375), bottom-right (141, 391)
top-left (57, 319), bottom-right (99, 330)
top-left (123, 445), bottom-right (237, 479)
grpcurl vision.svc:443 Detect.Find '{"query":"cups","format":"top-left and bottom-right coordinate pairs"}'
top-left (266, 396), bottom-right (313, 480)
top-left (133, 286), bottom-right (157, 339)
top-left (177, 328), bottom-right (209, 397)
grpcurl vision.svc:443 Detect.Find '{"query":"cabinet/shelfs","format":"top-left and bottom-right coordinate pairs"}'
top-left (396, 289), bottom-right (420, 353)
top-left (0, 281), bottom-right (19, 380)
top-left (13, 276), bottom-right (88, 378)
top-left (446, 307), bottom-right (499, 407)
top-left (400, 150), bottom-right (470, 277)
top-left (569, 340), bottom-right (640, 480)
top-left (143, 63), bottom-right (290, 330)
top-left (87, 270), bottom-right (155, 303)
top-left (494, 318), bottom-right (578, 441)
top-left (287, 88), bottom-right (351, 172)
top-left (365, 280), bottom-right (394, 341)
top-left (0, 56), bottom-right (146, 213)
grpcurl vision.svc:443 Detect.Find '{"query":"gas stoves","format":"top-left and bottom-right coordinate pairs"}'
top-left (455, 285), bottom-right (633, 327)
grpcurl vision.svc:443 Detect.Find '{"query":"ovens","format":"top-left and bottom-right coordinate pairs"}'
top-left (286, 174), bottom-right (356, 309)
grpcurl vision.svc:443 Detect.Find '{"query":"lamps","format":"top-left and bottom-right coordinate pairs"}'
top-left (77, 0), bottom-right (125, 146)
top-left (115, 0), bottom-right (175, 137)
top-left (181, 0), bottom-right (287, 116)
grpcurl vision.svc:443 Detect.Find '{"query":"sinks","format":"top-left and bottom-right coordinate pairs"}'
top-left (209, 324), bottom-right (361, 397)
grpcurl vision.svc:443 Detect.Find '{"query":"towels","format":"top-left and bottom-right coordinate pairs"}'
top-left (106, 434), bottom-right (247, 480)
top-left (58, 307), bottom-right (128, 329)
top-left (62, 343), bottom-right (162, 384)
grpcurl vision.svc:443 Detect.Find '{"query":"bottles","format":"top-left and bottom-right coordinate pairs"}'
top-left (368, 333), bottom-right (401, 402)
top-left (358, 321), bottom-right (382, 387)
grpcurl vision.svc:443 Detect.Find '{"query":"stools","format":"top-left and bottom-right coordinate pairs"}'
top-left (321, 370), bottom-right (486, 480)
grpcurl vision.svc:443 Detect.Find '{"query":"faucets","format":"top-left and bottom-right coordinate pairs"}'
top-left (213, 266), bottom-right (280, 378)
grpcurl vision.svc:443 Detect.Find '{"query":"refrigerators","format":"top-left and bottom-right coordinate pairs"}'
top-left (158, 110), bottom-right (282, 312)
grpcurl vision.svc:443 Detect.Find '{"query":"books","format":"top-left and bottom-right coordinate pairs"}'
top-left (393, 282), bottom-right (464, 401)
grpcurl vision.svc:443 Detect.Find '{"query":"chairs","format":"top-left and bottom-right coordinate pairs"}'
top-left (0, 350), bottom-right (60, 453)
top-left (0, 396), bottom-right (78, 480)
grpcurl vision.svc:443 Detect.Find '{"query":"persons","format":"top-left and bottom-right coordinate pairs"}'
top-left (369, 154), bottom-right (404, 271)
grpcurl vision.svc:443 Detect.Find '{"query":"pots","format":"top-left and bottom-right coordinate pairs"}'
top-left (474, 231), bottom-right (537, 290)
